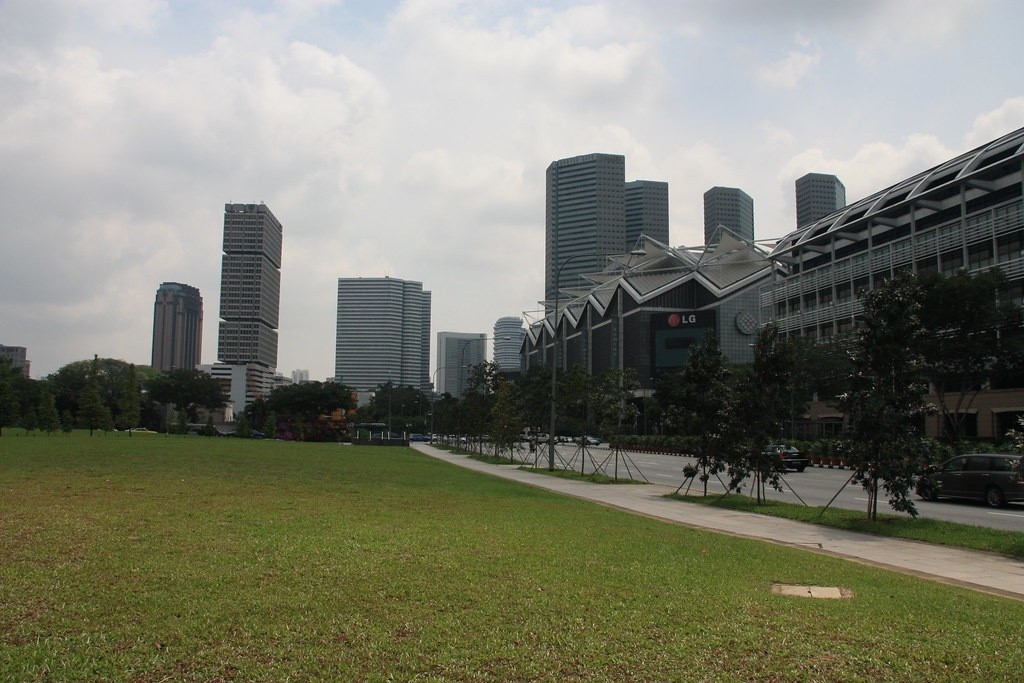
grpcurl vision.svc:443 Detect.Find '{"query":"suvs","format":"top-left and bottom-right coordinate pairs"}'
top-left (915, 453), bottom-right (1024, 509)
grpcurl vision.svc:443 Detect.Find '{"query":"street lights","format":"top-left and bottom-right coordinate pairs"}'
top-left (458, 336), bottom-right (511, 399)
top-left (429, 365), bottom-right (469, 444)
top-left (547, 249), bottom-right (648, 471)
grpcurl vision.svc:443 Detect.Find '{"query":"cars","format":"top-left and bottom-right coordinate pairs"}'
top-left (744, 444), bottom-right (809, 471)
top-left (125, 427), bottom-right (158, 434)
top-left (408, 431), bottom-right (490, 444)
top-left (519, 429), bottom-right (602, 446)
top-left (217, 429), bottom-right (265, 438)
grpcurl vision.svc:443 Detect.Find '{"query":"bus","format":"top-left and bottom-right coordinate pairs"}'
top-left (168, 423), bottom-right (216, 435)
